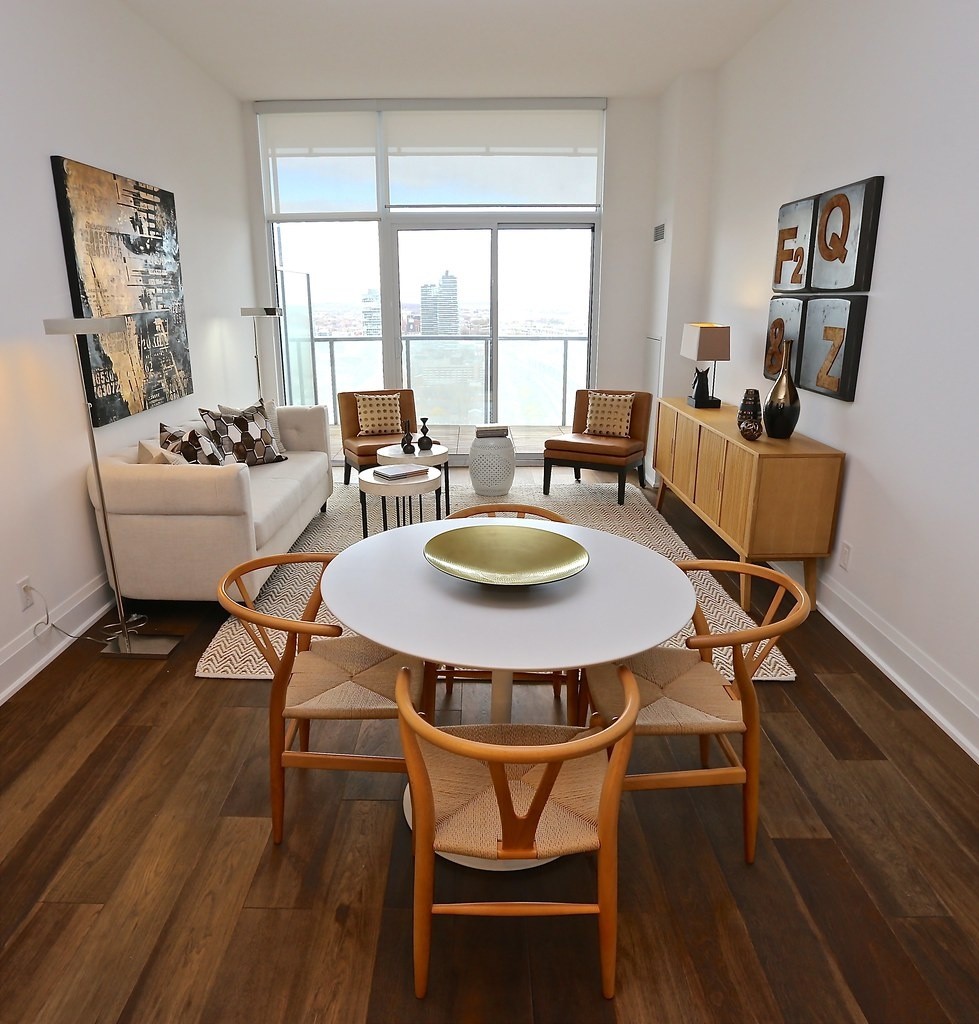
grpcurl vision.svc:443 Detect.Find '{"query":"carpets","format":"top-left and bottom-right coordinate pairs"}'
top-left (194, 483), bottom-right (797, 681)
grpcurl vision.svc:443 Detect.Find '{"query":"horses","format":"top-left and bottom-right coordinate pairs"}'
top-left (691, 367), bottom-right (710, 398)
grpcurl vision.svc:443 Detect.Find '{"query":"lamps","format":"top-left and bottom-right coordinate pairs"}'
top-left (679, 322), bottom-right (730, 408)
top-left (241, 307), bottom-right (283, 400)
top-left (42, 315), bottom-right (185, 660)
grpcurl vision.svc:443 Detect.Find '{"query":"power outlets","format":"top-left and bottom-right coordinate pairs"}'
top-left (17, 576), bottom-right (35, 611)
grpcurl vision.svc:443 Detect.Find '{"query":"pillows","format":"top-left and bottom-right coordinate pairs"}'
top-left (137, 399), bottom-right (288, 465)
top-left (582, 390), bottom-right (635, 438)
top-left (354, 392), bottom-right (405, 437)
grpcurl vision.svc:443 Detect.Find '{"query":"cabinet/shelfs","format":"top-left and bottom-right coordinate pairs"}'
top-left (652, 397), bottom-right (844, 611)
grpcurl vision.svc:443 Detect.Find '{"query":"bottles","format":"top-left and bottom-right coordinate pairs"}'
top-left (417, 418), bottom-right (432, 450)
top-left (737, 389), bottom-right (763, 429)
top-left (403, 420), bottom-right (415, 455)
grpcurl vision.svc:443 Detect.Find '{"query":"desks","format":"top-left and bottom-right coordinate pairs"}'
top-left (359, 464), bottom-right (442, 539)
top-left (320, 517), bottom-right (696, 727)
top-left (377, 442), bottom-right (450, 527)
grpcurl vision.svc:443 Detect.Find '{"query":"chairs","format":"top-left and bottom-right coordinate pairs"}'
top-left (442, 503), bottom-right (572, 699)
top-left (217, 552), bottom-right (437, 844)
top-left (396, 665), bottom-right (640, 998)
top-left (338, 389), bottom-right (441, 493)
top-left (543, 390), bottom-right (653, 505)
top-left (577, 559), bottom-right (811, 864)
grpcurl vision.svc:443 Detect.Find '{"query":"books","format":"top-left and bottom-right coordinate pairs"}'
top-left (373, 463), bottom-right (430, 481)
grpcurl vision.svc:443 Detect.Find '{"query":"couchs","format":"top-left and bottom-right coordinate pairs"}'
top-left (87, 403), bottom-right (334, 616)
